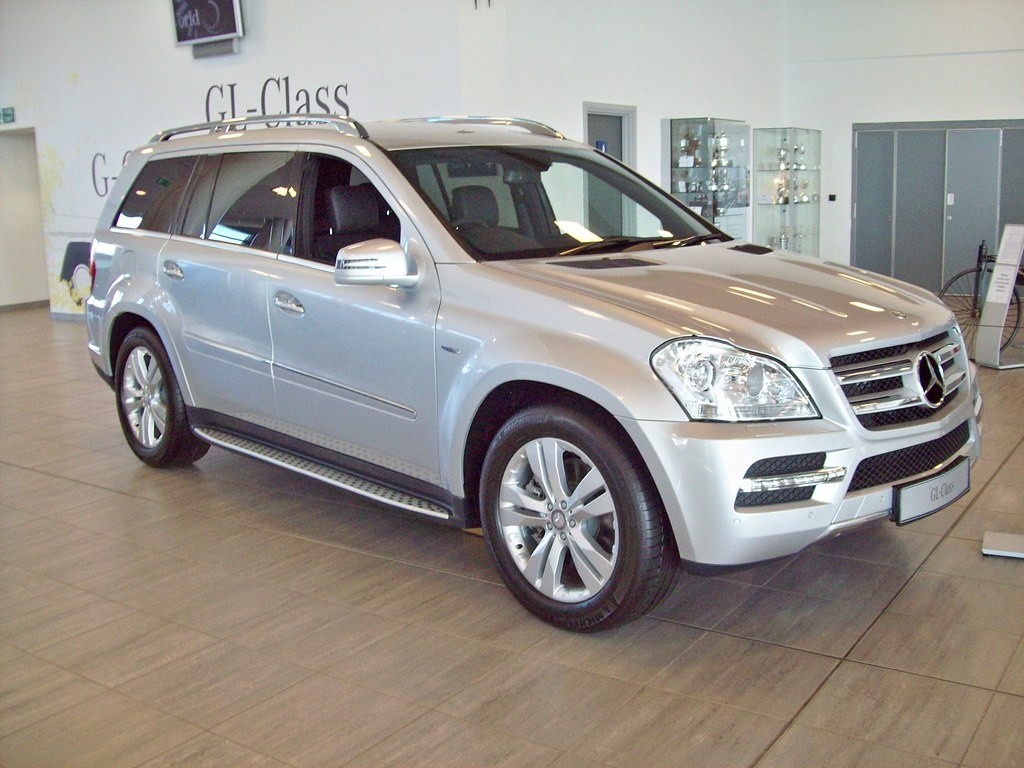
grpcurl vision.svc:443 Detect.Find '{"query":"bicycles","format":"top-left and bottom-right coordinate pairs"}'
top-left (941, 239), bottom-right (1023, 361)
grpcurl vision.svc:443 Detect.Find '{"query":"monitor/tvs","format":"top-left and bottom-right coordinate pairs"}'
top-left (170, 0), bottom-right (243, 47)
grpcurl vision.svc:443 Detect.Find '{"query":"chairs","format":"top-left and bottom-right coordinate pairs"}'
top-left (315, 182), bottom-right (547, 264)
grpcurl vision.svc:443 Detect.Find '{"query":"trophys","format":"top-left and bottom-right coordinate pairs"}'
top-left (774, 138), bottom-right (811, 252)
top-left (679, 130), bottom-right (728, 229)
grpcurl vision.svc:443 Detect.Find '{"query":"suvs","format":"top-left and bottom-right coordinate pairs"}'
top-left (82, 111), bottom-right (988, 633)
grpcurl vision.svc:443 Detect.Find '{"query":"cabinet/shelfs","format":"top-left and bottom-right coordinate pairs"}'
top-left (671, 117), bottom-right (750, 243)
top-left (753, 127), bottom-right (821, 257)
